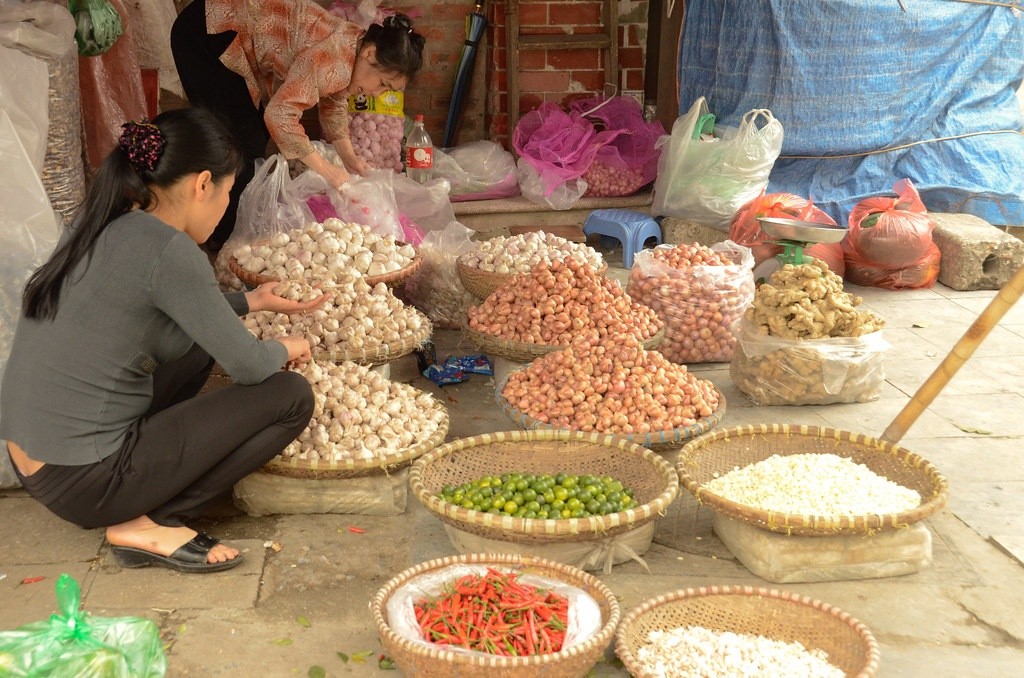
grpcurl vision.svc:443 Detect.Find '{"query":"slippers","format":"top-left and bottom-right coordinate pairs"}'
top-left (112, 526), bottom-right (243, 573)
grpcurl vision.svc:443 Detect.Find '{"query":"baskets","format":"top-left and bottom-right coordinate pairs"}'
top-left (230, 240), bottom-right (423, 293)
top-left (676, 423), bottom-right (949, 536)
top-left (456, 248), bottom-right (609, 301)
top-left (460, 305), bottom-right (666, 363)
top-left (258, 379), bottom-right (451, 480)
top-left (495, 364), bottom-right (727, 451)
top-left (312, 295), bottom-right (434, 368)
top-left (372, 553), bottom-right (619, 678)
top-left (611, 587), bottom-right (879, 678)
top-left (409, 429), bottom-right (681, 545)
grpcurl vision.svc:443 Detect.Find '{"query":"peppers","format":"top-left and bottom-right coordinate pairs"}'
top-left (413, 567), bottom-right (571, 657)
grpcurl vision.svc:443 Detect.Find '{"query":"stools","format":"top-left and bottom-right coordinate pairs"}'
top-left (583, 208), bottom-right (663, 271)
top-left (508, 222), bottom-right (588, 246)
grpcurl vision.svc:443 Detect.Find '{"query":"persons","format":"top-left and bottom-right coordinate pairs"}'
top-left (170, 0), bottom-right (428, 254)
top-left (0, 103), bottom-right (331, 573)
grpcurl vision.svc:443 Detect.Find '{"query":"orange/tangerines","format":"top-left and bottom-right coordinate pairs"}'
top-left (438, 471), bottom-right (639, 519)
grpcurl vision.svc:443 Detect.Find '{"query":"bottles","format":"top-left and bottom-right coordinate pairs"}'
top-left (406, 114), bottom-right (431, 186)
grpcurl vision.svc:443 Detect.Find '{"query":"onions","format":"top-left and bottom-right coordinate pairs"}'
top-left (627, 242), bottom-right (755, 363)
top-left (467, 260), bottom-right (718, 435)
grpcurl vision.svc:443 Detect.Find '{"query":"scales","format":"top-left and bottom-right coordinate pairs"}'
top-left (750, 216), bottom-right (849, 282)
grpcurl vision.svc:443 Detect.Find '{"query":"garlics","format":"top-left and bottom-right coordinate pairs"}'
top-left (462, 229), bottom-right (603, 273)
top-left (215, 110), bottom-right (444, 461)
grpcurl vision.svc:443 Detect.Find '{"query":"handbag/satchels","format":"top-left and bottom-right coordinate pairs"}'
top-left (651, 95), bottom-right (786, 234)
top-left (850, 178), bottom-right (933, 268)
top-left (210, 136), bottom-right (483, 329)
top-left (839, 228), bottom-right (942, 290)
top-left (627, 239), bottom-right (755, 366)
top-left (729, 191), bottom-right (847, 294)
top-left (729, 308), bottom-right (891, 405)
top-left (1, 574), bottom-right (166, 678)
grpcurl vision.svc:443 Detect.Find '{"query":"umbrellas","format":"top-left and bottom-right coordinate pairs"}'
top-left (441, 0), bottom-right (489, 154)
